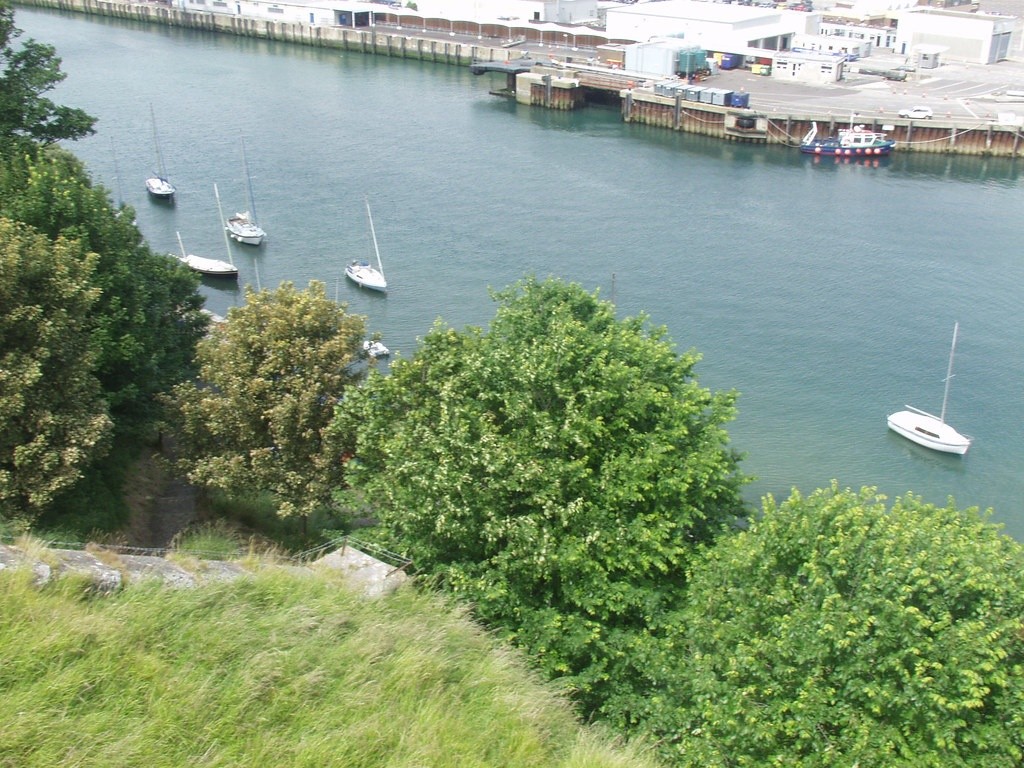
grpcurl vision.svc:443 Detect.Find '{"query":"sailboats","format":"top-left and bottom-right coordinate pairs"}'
top-left (343, 200), bottom-right (390, 296)
top-left (887, 320), bottom-right (973, 457)
top-left (224, 136), bottom-right (267, 247)
top-left (168, 184), bottom-right (238, 276)
top-left (106, 136), bottom-right (137, 228)
top-left (144, 103), bottom-right (176, 198)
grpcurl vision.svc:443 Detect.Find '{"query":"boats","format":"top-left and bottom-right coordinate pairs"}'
top-left (199, 307), bottom-right (229, 325)
top-left (798, 120), bottom-right (898, 158)
top-left (359, 339), bottom-right (390, 358)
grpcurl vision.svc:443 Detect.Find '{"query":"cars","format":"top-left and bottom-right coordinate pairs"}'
top-left (898, 106), bottom-right (932, 119)
